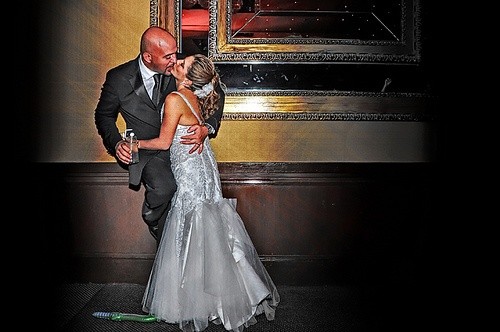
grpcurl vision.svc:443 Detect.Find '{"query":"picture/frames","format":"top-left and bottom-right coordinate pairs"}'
top-left (151, 1), bottom-right (499, 121)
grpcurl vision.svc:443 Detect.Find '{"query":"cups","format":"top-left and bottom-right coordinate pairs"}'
top-left (126, 133), bottom-right (140, 163)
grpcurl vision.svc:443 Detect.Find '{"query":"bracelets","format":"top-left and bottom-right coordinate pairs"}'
top-left (137, 139), bottom-right (140, 149)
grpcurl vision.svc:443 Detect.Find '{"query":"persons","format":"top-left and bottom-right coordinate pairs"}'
top-left (94, 26), bottom-right (226, 247)
top-left (123, 52), bottom-right (280, 331)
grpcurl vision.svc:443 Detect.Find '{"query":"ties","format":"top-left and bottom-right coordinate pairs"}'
top-left (152, 74), bottom-right (163, 109)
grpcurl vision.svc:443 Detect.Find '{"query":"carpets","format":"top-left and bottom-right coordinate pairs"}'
top-left (63, 282), bottom-right (498, 332)
